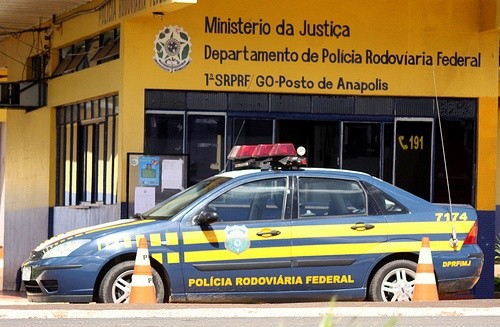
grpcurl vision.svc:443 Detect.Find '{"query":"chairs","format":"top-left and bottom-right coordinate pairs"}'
top-left (326, 184), bottom-right (348, 216)
top-left (350, 189), bottom-right (366, 214)
top-left (273, 188), bottom-right (284, 208)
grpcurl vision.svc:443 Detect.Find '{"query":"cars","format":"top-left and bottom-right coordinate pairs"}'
top-left (21, 143), bottom-right (486, 304)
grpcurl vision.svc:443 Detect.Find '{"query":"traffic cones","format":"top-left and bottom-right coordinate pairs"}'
top-left (128, 236), bottom-right (159, 304)
top-left (411, 235), bottom-right (441, 303)
